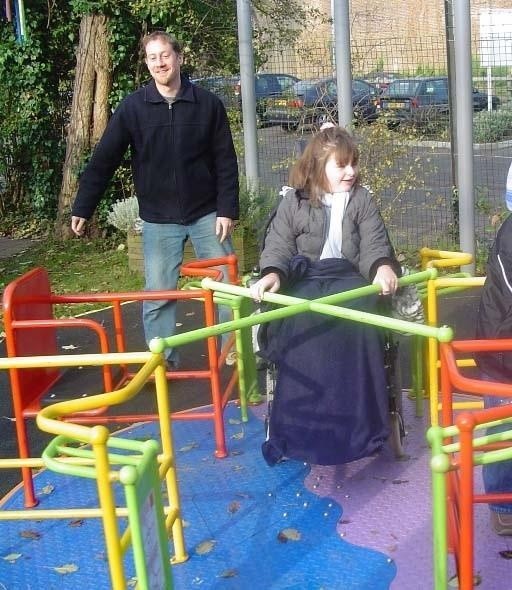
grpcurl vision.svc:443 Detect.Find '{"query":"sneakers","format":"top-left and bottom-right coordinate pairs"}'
top-left (226, 350), bottom-right (266, 371)
top-left (489, 510), bottom-right (511, 534)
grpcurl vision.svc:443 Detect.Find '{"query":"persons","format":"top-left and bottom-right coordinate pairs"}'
top-left (472, 168), bottom-right (510, 540)
top-left (71, 29), bottom-right (250, 370)
top-left (243, 126), bottom-right (407, 469)
